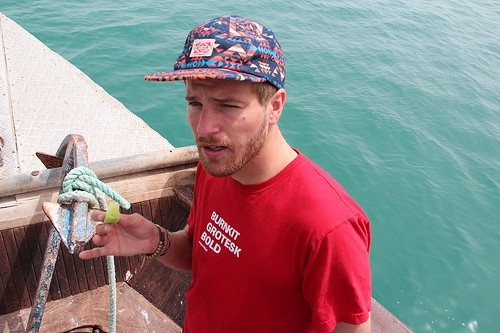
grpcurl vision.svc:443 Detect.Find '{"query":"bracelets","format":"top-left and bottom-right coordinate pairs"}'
top-left (140, 224), bottom-right (171, 260)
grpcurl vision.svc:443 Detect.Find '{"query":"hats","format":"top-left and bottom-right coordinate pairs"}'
top-left (144, 15), bottom-right (286, 89)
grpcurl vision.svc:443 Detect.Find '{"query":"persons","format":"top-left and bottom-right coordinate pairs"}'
top-left (79, 15), bottom-right (372, 333)
